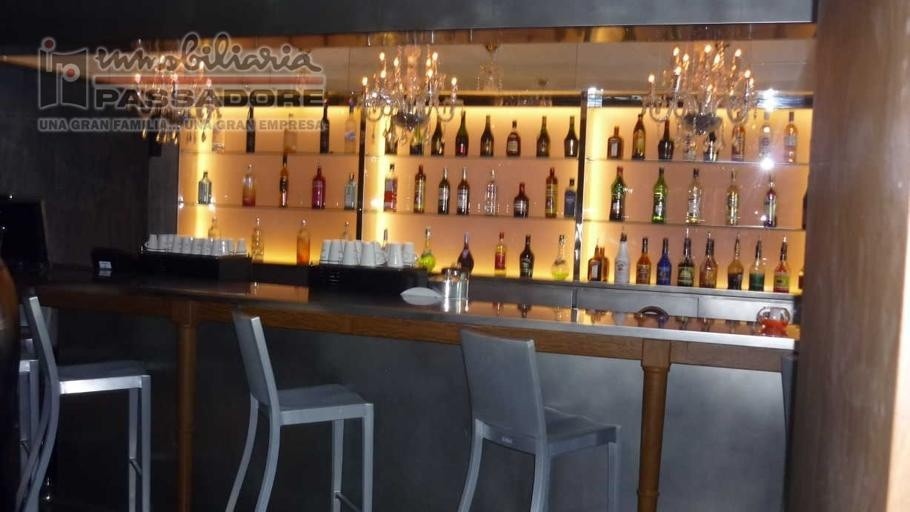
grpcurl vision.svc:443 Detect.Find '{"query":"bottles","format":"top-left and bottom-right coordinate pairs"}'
top-left (551, 234), bottom-right (569, 282)
top-left (418, 224), bottom-right (435, 270)
top-left (197, 172), bottom-right (211, 205)
top-left (518, 234), bottom-right (535, 278)
top-left (251, 218), bottom-right (264, 257)
top-left (208, 216), bottom-right (221, 239)
top-left (383, 114), bottom-right (578, 159)
top-left (242, 164), bottom-right (255, 205)
top-left (583, 234), bottom-right (791, 293)
top-left (296, 219), bottom-right (310, 266)
top-left (345, 101), bottom-right (357, 154)
top-left (383, 230), bottom-right (389, 246)
top-left (340, 222), bottom-right (353, 242)
top-left (278, 155), bottom-right (288, 207)
top-left (343, 174), bottom-right (355, 209)
top-left (246, 102), bottom-right (255, 152)
top-left (607, 110), bottom-right (799, 163)
top-left (283, 114), bottom-right (296, 151)
top-left (493, 231), bottom-right (506, 273)
top-left (491, 297), bottom-right (568, 321)
top-left (382, 161), bottom-right (575, 218)
top-left (310, 165), bottom-right (326, 207)
top-left (320, 101), bottom-right (329, 152)
top-left (586, 307), bottom-right (760, 336)
top-left (457, 232), bottom-right (474, 274)
top-left (610, 167), bottom-right (780, 226)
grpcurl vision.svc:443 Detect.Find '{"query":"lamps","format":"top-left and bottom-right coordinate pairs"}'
top-left (647, 41), bottom-right (762, 160)
top-left (131, 50), bottom-right (222, 146)
top-left (360, 43), bottom-right (459, 149)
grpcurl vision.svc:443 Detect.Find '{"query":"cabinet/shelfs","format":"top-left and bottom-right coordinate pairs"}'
top-left (145, 93), bottom-right (813, 330)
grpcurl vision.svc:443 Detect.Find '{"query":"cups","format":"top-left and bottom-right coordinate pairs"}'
top-left (443, 267), bottom-right (469, 300)
top-left (319, 238), bottom-right (417, 267)
top-left (149, 235), bottom-right (247, 257)
top-left (756, 306), bottom-right (790, 329)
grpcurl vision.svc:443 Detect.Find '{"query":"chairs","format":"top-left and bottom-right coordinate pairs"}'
top-left (226, 314), bottom-right (374, 512)
top-left (14, 288), bottom-right (152, 511)
top-left (459, 327), bottom-right (619, 511)
